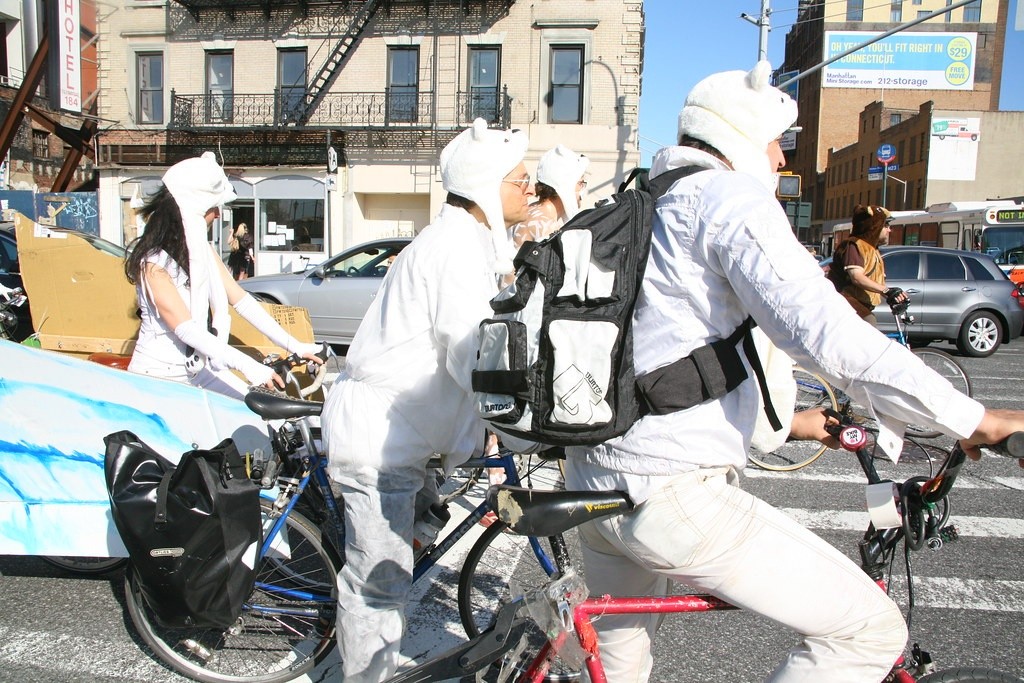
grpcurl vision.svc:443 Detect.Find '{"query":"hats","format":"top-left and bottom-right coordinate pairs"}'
top-left (537, 147), bottom-right (591, 221)
top-left (678, 60), bottom-right (798, 171)
top-left (162, 151), bottom-right (239, 372)
top-left (439, 117), bottom-right (527, 289)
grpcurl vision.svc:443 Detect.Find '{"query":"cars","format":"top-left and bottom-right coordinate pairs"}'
top-left (818, 247), bottom-right (1022, 357)
top-left (0, 220), bottom-right (138, 315)
top-left (230, 235), bottom-right (545, 372)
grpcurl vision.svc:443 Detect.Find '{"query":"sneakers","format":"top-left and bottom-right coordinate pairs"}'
top-left (480, 510), bottom-right (519, 535)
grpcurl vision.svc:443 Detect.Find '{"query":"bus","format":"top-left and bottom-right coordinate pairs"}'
top-left (831, 198), bottom-right (1024, 307)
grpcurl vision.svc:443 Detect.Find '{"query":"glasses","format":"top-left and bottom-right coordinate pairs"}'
top-left (578, 181), bottom-right (587, 189)
top-left (882, 222), bottom-right (889, 228)
top-left (501, 177), bottom-right (531, 191)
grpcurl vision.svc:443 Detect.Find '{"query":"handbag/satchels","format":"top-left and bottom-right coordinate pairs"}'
top-left (105, 431), bottom-right (261, 629)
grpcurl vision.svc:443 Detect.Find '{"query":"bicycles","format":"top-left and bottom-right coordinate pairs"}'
top-left (123, 386), bottom-right (560, 683)
top-left (478, 402), bottom-right (1022, 683)
top-left (732, 282), bottom-right (978, 472)
top-left (38, 339), bottom-right (491, 577)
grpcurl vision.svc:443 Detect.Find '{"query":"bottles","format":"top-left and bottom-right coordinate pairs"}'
top-left (412, 502), bottom-right (451, 562)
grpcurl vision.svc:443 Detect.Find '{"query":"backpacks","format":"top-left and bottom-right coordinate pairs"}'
top-left (829, 246), bottom-right (877, 289)
top-left (470, 165), bottom-right (758, 447)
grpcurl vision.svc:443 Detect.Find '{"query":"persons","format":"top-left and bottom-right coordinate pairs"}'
top-left (318, 113), bottom-right (538, 683)
top-left (826, 202), bottom-right (908, 326)
top-left (294, 226), bottom-right (311, 244)
top-left (564, 54), bottom-right (1024, 683)
top-left (126, 151), bottom-right (328, 400)
top-left (479, 143), bottom-right (590, 532)
top-left (228, 223), bottom-right (254, 281)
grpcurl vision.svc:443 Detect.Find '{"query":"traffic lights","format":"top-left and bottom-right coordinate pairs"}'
top-left (778, 173), bottom-right (802, 199)
